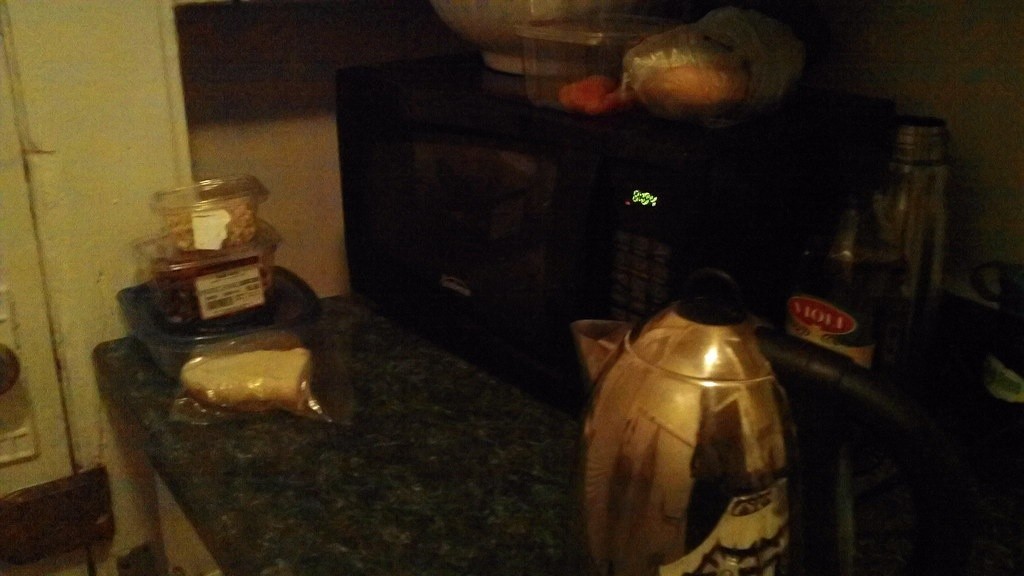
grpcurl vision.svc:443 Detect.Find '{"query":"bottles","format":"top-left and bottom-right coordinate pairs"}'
top-left (873, 114), bottom-right (948, 303)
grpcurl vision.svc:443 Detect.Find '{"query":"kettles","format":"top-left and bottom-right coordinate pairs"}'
top-left (566, 268), bottom-right (934, 576)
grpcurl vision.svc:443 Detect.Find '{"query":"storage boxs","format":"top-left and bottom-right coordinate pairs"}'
top-left (513, 10), bottom-right (673, 116)
top-left (116, 173), bottom-right (323, 380)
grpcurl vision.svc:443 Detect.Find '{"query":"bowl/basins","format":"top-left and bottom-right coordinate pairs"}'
top-left (428, 0), bottom-right (660, 76)
